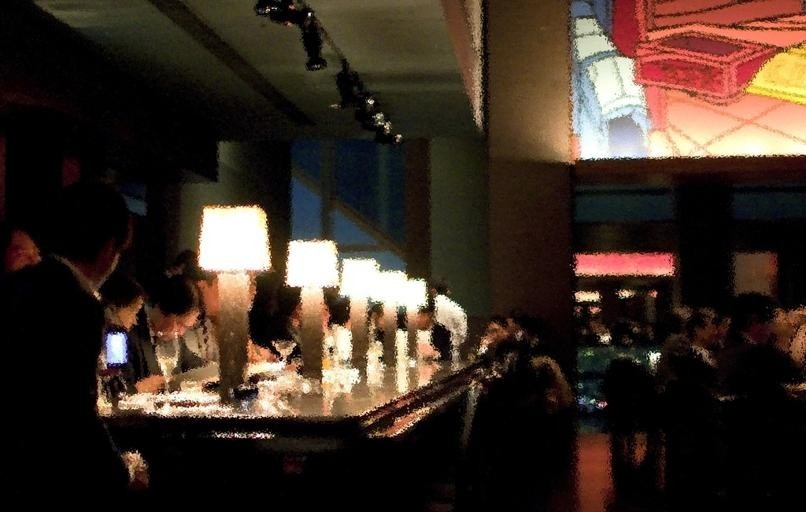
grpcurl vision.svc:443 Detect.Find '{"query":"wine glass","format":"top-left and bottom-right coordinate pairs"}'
top-left (271, 340), bottom-right (298, 367)
top-left (153, 330), bottom-right (178, 415)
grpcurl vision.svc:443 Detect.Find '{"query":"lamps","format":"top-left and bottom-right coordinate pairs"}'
top-left (255, 2), bottom-right (402, 149)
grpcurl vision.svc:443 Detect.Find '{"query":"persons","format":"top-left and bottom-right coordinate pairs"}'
top-left (2, 176), bottom-right (804, 510)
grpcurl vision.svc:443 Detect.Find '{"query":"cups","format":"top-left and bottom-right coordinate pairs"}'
top-left (322, 348), bottom-right (333, 370)
